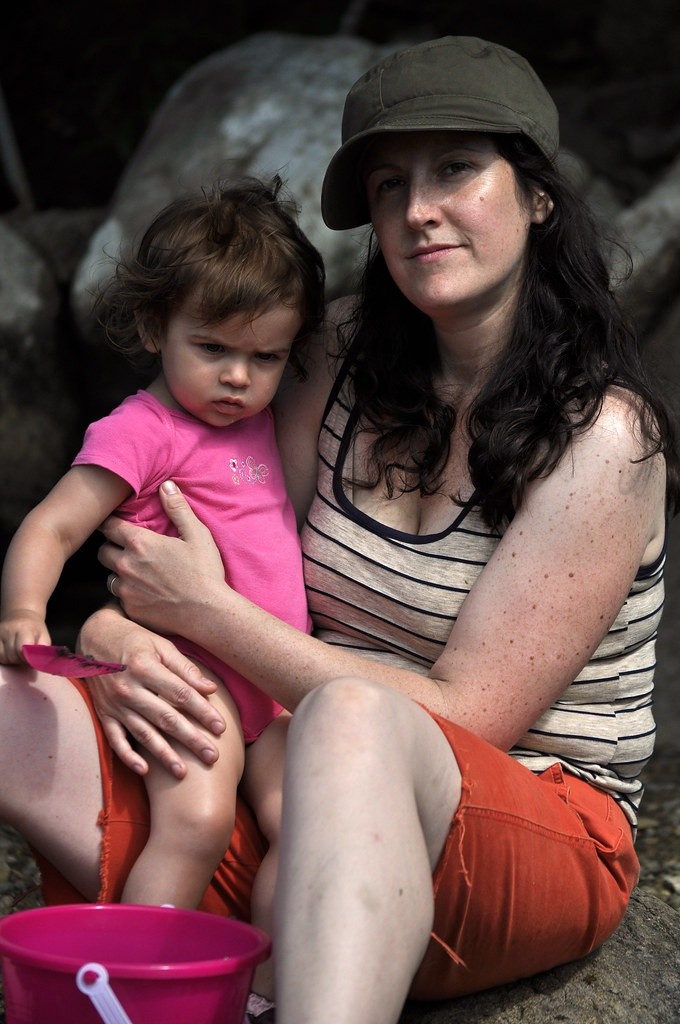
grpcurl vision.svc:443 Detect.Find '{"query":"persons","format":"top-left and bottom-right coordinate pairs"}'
top-left (76, 36), bottom-right (668, 1023)
top-left (0, 171), bottom-right (331, 1023)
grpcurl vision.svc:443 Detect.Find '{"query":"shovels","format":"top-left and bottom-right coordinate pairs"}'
top-left (23, 643), bottom-right (128, 679)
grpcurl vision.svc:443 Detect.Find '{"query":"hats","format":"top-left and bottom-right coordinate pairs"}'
top-left (321, 35), bottom-right (562, 231)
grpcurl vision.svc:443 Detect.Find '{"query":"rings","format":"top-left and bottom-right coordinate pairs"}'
top-left (110, 577), bottom-right (118, 598)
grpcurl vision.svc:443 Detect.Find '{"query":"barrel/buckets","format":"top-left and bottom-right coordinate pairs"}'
top-left (0, 903), bottom-right (272, 1024)
